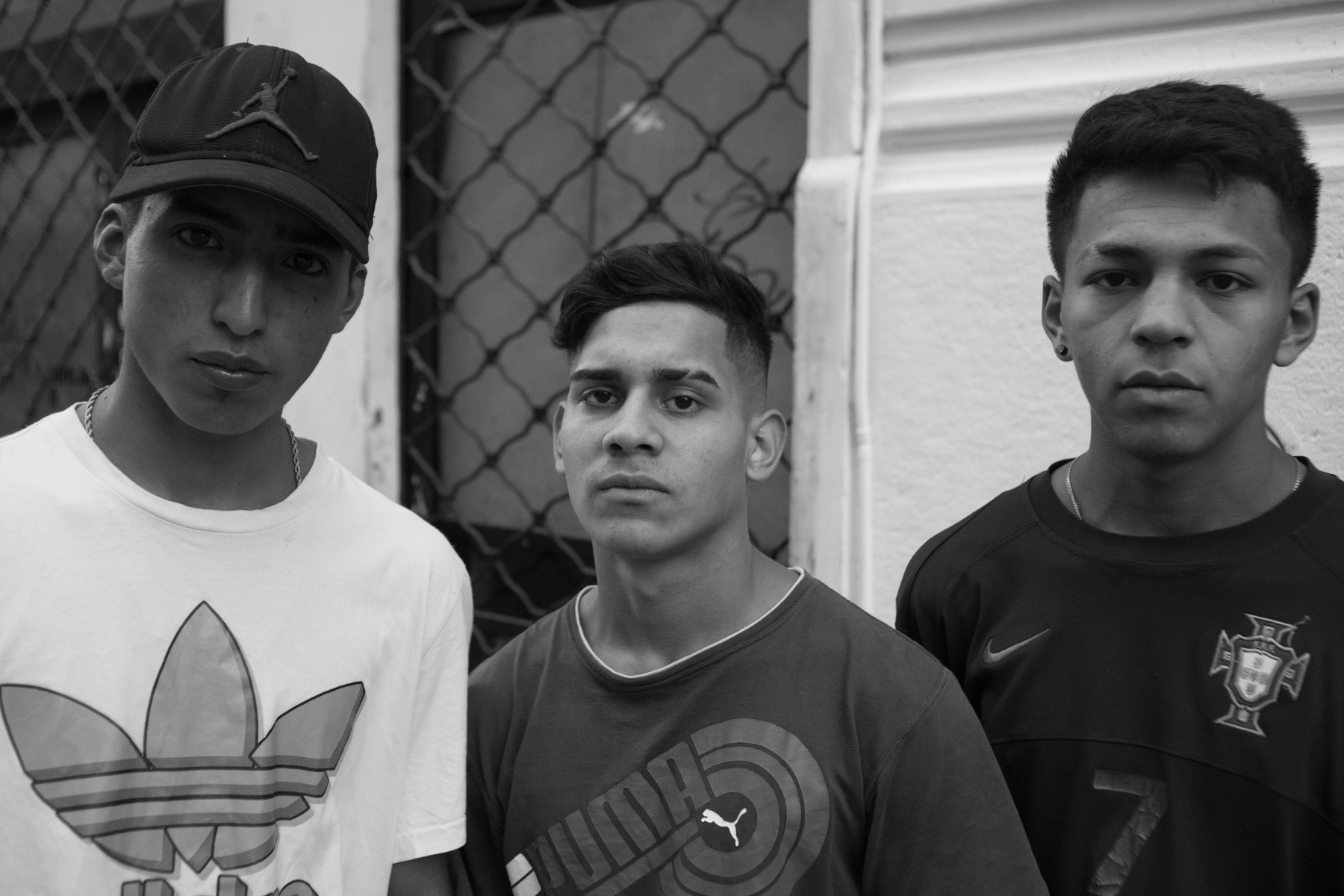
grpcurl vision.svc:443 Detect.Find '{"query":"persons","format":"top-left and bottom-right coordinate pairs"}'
top-left (0, 41), bottom-right (474, 896)
top-left (894, 82), bottom-right (1344, 896)
top-left (447, 242), bottom-right (1052, 896)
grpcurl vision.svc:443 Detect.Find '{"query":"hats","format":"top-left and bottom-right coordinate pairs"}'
top-left (110, 43), bottom-right (379, 265)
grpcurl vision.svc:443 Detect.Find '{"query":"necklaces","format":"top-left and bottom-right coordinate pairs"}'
top-left (1066, 452), bottom-right (1300, 520)
top-left (84, 385), bottom-right (301, 486)
